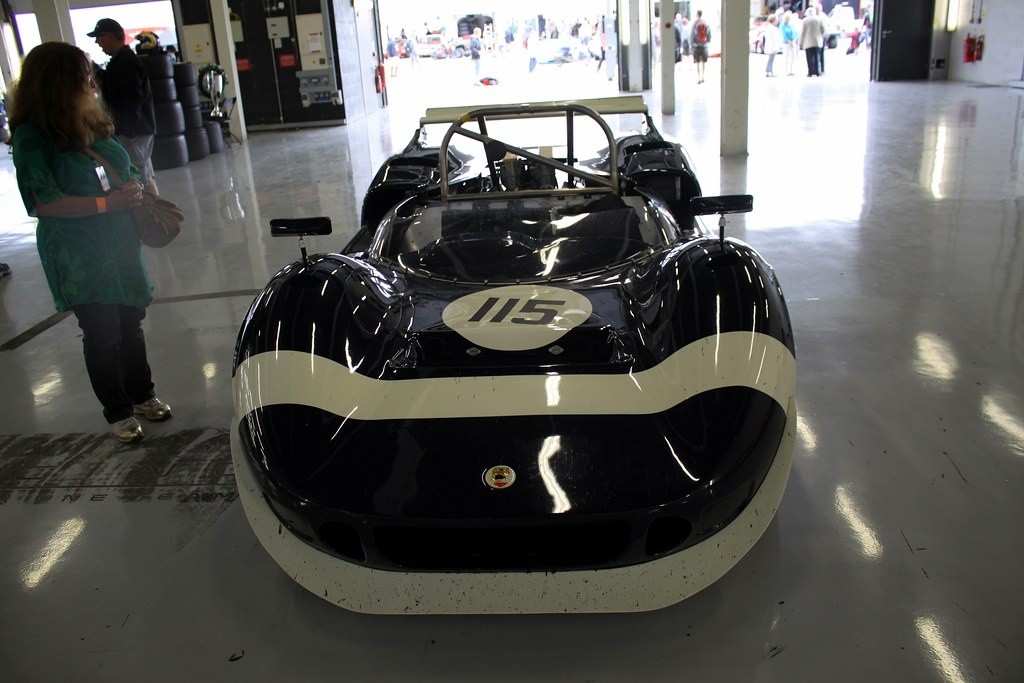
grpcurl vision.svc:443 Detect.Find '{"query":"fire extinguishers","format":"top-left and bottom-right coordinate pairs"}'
top-left (975, 34), bottom-right (984, 60)
top-left (963, 32), bottom-right (975, 63)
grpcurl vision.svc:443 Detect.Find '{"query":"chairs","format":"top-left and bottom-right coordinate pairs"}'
top-left (211, 97), bottom-right (242, 149)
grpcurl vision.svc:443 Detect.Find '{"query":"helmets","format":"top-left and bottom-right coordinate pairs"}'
top-left (135, 32), bottom-right (159, 55)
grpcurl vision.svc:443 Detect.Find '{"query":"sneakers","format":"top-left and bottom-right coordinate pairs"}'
top-left (132, 396), bottom-right (172, 420)
top-left (108, 416), bottom-right (143, 442)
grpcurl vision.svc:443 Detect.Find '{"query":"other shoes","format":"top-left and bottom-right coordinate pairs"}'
top-left (0, 263), bottom-right (10, 277)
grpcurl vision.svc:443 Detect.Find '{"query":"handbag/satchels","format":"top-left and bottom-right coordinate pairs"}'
top-left (131, 192), bottom-right (185, 249)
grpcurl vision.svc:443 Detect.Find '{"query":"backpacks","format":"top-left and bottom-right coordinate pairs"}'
top-left (695, 19), bottom-right (708, 42)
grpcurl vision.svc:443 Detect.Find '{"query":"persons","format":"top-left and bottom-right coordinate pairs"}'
top-left (387, 18), bottom-right (606, 85)
top-left (763, 2), bottom-right (870, 77)
top-left (674, 9), bottom-right (711, 84)
top-left (87, 18), bottom-right (159, 196)
top-left (0, 41), bottom-right (174, 442)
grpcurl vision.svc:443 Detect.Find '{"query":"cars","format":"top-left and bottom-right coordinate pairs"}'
top-left (416, 33), bottom-right (499, 59)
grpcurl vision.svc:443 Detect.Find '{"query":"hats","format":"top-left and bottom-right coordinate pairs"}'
top-left (87, 18), bottom-right (125, 37)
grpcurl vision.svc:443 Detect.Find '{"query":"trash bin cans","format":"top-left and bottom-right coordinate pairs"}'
top-left (930, 56), bottom-right (947, 80)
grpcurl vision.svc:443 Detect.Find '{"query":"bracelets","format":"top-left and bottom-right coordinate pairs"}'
top-left (94, 196), bottom-right (107, 215)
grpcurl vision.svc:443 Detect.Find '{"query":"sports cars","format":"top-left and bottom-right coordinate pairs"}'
top-left (227, 93), bottom-right (800, 617)
